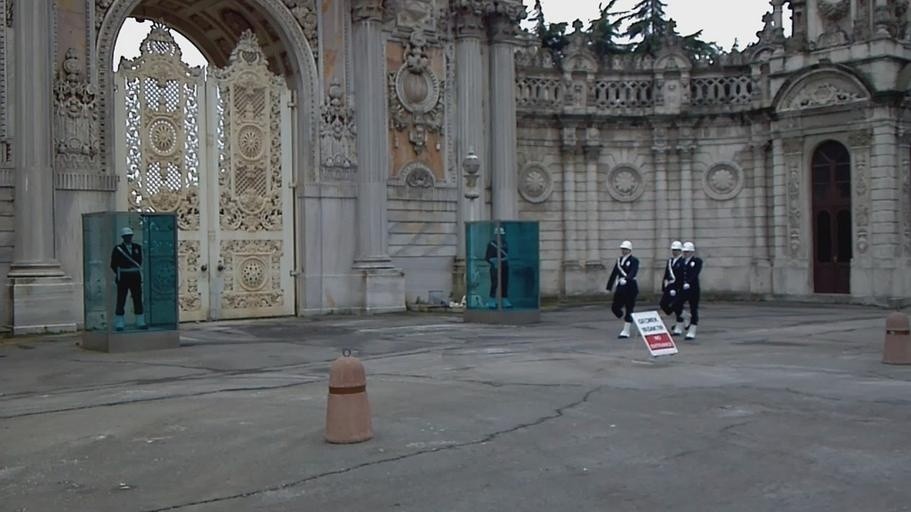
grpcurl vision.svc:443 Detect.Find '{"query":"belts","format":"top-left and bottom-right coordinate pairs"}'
top-left (119, 268), bottom-right (140, 271)
top-left (492, 257), bottom-right (506, 261)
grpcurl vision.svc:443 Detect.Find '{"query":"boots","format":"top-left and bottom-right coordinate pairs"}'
top-left (619, 322), bottom-right (631, 338)
top-left (673, 309), bottom-right (697, 339)
top-left (135, 315), bottom-right (147, 329)
top-left (115, 314), bottom-right (124, 331)
top-left (501, 297), bottom-right (512, 307)
top-left (488, 296), bottom-right (496, 308)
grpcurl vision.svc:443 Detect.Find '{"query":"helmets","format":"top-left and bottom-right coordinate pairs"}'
top-left (494, 227), bottom-right (505, 235)
top-left (671, 241), bottom-right (682, 250)
top-left (120, 227), bottom-right (133, 236)
top-left (682, 242), bottom-right (695, 252)
top-left (619, 240), bottom-right (632, 251)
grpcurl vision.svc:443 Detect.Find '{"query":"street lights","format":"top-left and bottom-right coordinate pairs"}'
top-left (461, 151), bottom-right (480, 223)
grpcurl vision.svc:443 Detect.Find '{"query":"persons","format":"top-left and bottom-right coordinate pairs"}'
top-left (660, 241), bottom-right (685, 335)
top-left (671, 242), bottom-right (703, 339)
top-left (111, 227), bottom-right (149, 330)
top-left (486, 226), bottom-right (513, 309)
top-left (605, 240), bottom-right (639, 338)
